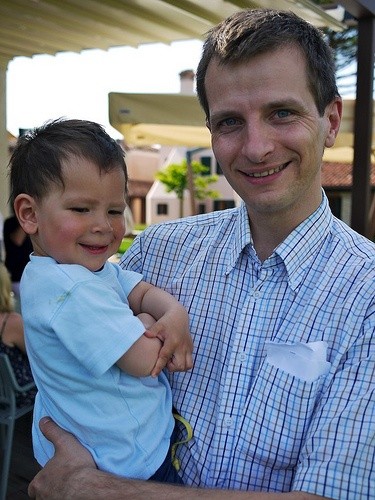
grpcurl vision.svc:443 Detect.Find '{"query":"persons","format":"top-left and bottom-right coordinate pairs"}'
top-left (26, 10), bottom-right (375, 500)
top-left (0, 215), bottom-right (38, 407)
top-left (8, 120), bottom-right (197, 485)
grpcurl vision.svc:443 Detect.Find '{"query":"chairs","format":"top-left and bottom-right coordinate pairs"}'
top-left (0, 354), bottom-right (37, 500)
